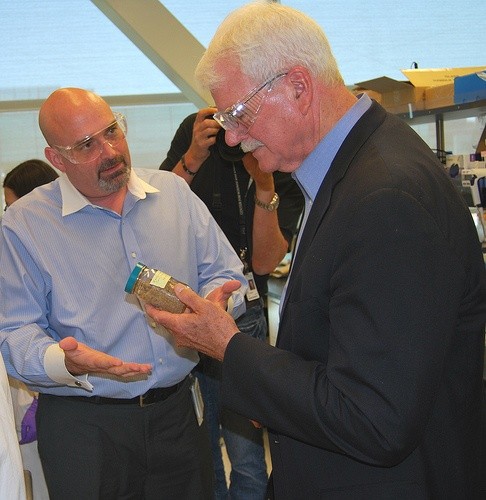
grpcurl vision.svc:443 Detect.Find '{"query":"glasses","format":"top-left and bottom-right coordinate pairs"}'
top-left (50, 111), bottom-right (129, 163)
top-left (212, 72), bottom-right (289, 135)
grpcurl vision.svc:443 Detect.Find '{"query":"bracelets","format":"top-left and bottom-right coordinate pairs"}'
top-left (181, 154), bottom-right (198, 177)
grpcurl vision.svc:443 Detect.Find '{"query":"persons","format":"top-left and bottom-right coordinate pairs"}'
top-left (4, 159), bottom-right (60, 212)
top-left (158, 105), bottom-right (305, 500)
top-left (0, 88), bottom-right (248, 500)
top-left (144, 1), bottom-right (485, 500)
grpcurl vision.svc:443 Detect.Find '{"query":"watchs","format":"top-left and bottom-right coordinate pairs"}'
top-left (253, 191), bottom-right (279, 211)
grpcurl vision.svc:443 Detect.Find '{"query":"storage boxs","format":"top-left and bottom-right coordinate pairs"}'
top-left (454, 68), bottom-right (486, 104)
top-left (424, 82), bottom-right (455, 108)
top-left (354, 65), bottom-right (486, 117)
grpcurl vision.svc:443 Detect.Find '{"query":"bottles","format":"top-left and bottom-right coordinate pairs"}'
top-left (124, 262), bottom-right (190, 314)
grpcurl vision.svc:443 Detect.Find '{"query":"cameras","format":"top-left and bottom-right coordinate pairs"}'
top-left (215, 129), bottom-right (247, 162)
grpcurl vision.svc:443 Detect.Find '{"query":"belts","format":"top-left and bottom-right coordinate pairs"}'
top-left (60, 376), bottom-right (185, 406)
